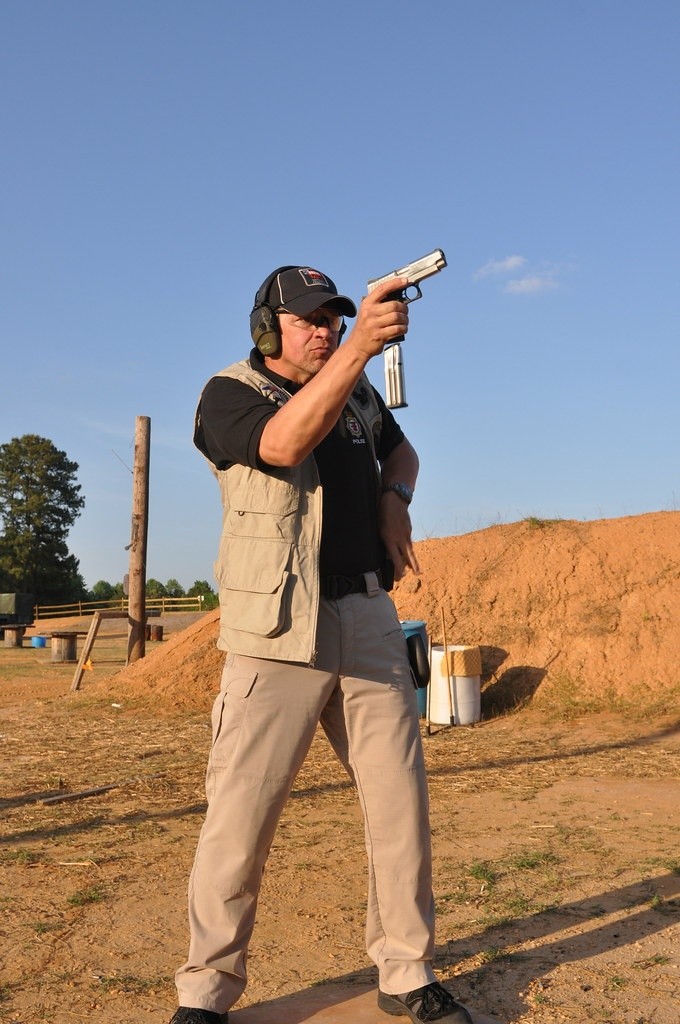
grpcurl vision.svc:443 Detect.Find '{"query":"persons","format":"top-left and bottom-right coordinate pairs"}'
top-left (168, 266), bottom-right (475, 1024)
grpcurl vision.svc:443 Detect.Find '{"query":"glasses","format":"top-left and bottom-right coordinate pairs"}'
top-left (270, 307), bottom-right (344, 332)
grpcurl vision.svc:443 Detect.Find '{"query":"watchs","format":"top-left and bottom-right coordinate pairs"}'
top-left (382, 482), bottom-right (413, 504)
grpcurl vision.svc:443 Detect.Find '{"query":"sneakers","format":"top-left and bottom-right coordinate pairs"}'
top-left (378, 983), bottom-right (494, 1024)
top-left (168, 1007), bottom-right (228, 1024)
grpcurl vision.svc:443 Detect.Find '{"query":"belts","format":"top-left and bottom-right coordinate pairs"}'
top-left (319, 570), bottom-right (384, 600)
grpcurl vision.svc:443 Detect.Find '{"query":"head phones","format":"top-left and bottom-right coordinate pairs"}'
top-left (250, 266), bottom-right (347, 356)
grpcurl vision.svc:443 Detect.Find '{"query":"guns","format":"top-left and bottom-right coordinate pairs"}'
top-left (364, 246), bottom-right (448, 344)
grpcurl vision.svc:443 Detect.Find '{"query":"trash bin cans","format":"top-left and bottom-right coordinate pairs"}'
top-left (399, 620), bottom-right (432, 718)
top-left (425, 645), bottom-right (482, 725)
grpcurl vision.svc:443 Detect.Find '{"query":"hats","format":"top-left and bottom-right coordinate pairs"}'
top-left (255, 268), bottom-right (357, 318)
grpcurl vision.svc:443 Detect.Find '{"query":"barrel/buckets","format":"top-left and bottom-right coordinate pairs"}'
top-left (145, 624), bottom-right (151, 641)
top-left (400, 621), bottom-right (429, 718)
top-left (426, 645), bottom-right (481, 725)
top-left (152, 625), bottom-right (163, 641)
top-left (32, 637), bottom-right (45, 648)
